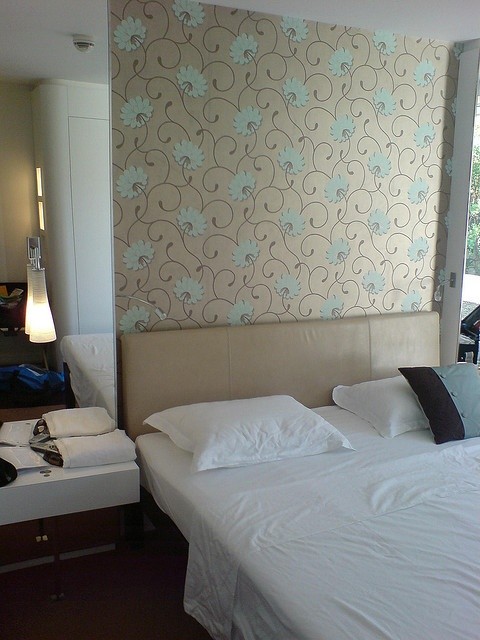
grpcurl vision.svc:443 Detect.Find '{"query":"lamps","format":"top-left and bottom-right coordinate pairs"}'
top-left (26, 233), bottom-right (60, 345)
top-left (23, 238), bottom-right (34, 333)
top-left (115, 294), bottom-right (167, 320)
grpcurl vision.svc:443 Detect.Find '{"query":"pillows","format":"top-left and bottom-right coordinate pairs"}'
top-left (332, 376), bottom-right (429, 440)
top-left (143, 395), bottom-right (355, 475)
top-left (396, 363), bottom-right (480, 444)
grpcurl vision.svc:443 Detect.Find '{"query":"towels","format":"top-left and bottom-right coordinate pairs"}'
top-left (52, 428), bottom-right (138, 469)
top-left (41, 407), bottom-right (117, 439)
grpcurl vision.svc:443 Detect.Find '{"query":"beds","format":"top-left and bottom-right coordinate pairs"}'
top-left (120, 313), bottom-right (480, 638)
top-left (58, 331), bottom-right (115, 415)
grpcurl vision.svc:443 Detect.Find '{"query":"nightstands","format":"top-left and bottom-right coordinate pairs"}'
top-left (1, 446), bottom-right (144, 592)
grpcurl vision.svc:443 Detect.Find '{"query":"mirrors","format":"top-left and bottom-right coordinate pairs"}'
top-left (2, 0), bottom-right (118, 445)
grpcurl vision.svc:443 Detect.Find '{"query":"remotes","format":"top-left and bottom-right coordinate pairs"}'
top-left (30, 442), bottom-right (60, 455)
top-left (29, 433), bottom-right (49, 443)
